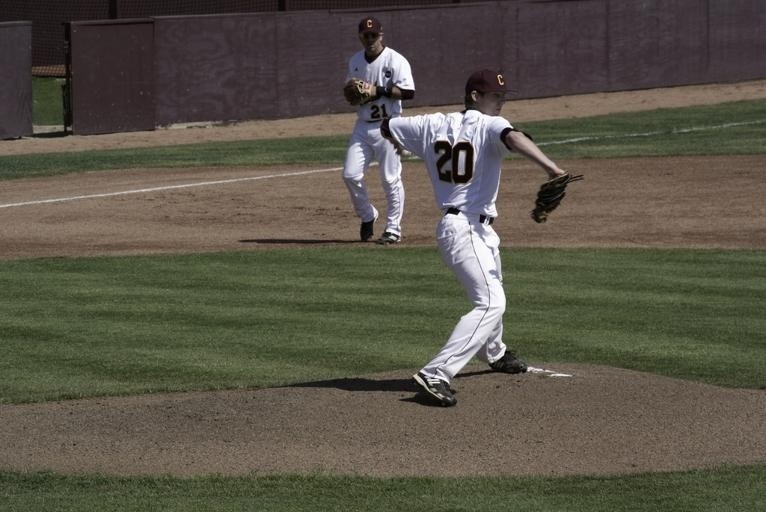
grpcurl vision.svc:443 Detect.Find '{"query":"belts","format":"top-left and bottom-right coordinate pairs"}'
top-left (445, 207), bottom-right (494, 225)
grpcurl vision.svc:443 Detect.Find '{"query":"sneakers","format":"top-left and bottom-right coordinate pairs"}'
top-left (360, 209), bottom-right (379, 240)
top-left (412, 372), bottom-right (457, 404)
top-left (377, 232), bottom-right (401, 245)
top-left (489, 350), bottom-right (527, 373)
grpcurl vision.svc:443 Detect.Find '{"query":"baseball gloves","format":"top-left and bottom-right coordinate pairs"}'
top-left (531, 170), bottom-right (585, 224)
top-left (344, 78), bottom-right (372, 105)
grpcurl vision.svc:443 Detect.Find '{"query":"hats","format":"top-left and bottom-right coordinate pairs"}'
top-left (466, 70), bottom-right (519, 97)
top-left (359, 16), bottom-right (382, 33)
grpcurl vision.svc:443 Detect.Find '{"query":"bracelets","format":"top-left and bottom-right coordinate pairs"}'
top-left (376, 86), bottom-right (392, 98)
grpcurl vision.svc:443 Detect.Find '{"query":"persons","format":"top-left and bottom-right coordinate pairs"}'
top-left (380, 71), bottom-right (570, 405)
top-left (342, 16), bottom-right (416, 245)
